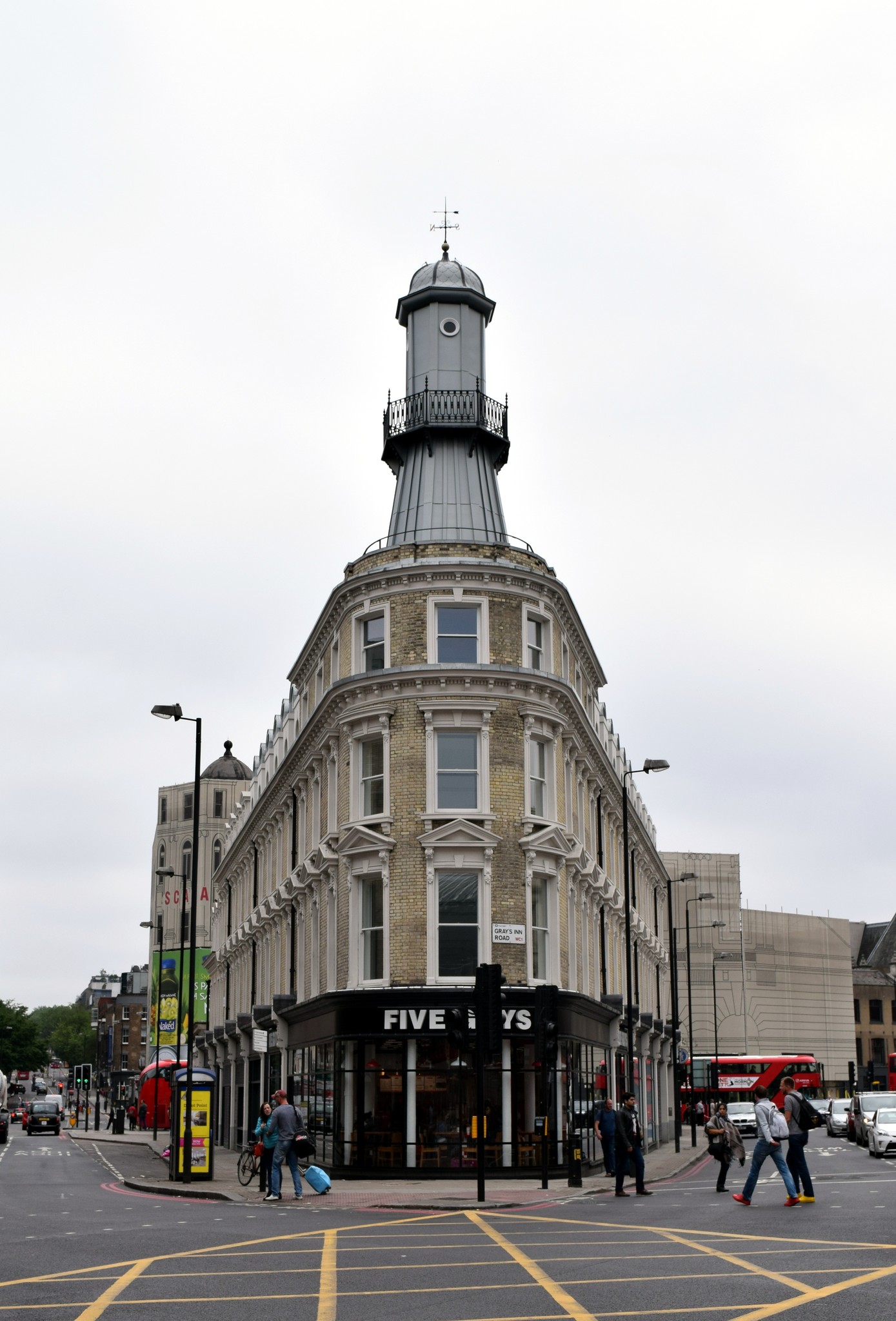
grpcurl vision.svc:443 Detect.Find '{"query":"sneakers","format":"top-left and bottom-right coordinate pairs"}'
top-left (293, 1195), bottom-right (303, 1200)
top-left (265, 1194), bottom-right (279, 1200)
top-left (784, 1197), bottom-right (800, 1206)
top-left (732, 1194), bottom-right (751, 1205)
top-left (786, 1192), bottom-right (802, 1199)
top-left (799, 1195), bottom-right (815, 1203)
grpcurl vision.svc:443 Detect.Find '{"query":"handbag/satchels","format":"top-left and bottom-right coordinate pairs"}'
top-left (293, 1130), bottom-right (316, 1157)
top-left (254, 1142), bottom-right (264, 1156)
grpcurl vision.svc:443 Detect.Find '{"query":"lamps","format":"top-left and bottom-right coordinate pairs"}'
top-left (450, 1049), bottom-right (467, 1066)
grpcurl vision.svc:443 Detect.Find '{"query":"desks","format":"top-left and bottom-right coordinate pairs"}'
top-left (520, 1131), bottom-right (538, 1166)
top-left (434, 1132), bottom-right (459, 1167)
top-left (365, 1131), bottom-right (391, 1142)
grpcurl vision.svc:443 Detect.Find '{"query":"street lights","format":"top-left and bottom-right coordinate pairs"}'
top-left (672, 920), bottom-right (726, 1136)
top-left (666, 872), bottom-right (699, 1153)
top-left (139, 920), bottom-right (164, 1141)
top-left (621, 758), bottom-right (671, 1093)
top-left (150, 703), bottom-right (203, 1183)
top-left (685, 891), bottom-right (716, 1147)
top-left (154, 865), bottom-right (188, 1065)
top-left (712, 952), bottom-right (733, 1107)
top-left (95, 1019), bottom-right (102, 1130)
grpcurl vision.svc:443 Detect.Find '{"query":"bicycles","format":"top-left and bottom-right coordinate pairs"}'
top-left (237, 1140), bottom-right (270, 1187)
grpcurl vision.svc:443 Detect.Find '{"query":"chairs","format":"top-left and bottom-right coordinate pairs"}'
top-left (514, 1134), bottom-right (535, 1168)
top-left (461, 1138), bottom-right (478, 1161)
top-left (377, 1132), bottom-right (402, 1168)
top-left (420, 1134), bottom-right (441, 1168)
top-left (485, 1132), bottom-right (502, 1167)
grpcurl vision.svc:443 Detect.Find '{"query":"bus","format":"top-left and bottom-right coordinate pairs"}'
top-left (136, 1059), bottom-right (188, 1129)
top-left (596, 1055), bottom-right (827, 1123)
top-left (888, 1053), bottom-right (896, 1092)
top-left (50, 1058), bottom-right (60, 1068)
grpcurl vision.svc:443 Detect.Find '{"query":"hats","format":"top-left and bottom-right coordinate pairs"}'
top-left (271, 1090), bottom-right (287, 1098)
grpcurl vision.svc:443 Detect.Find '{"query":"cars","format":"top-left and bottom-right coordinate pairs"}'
top-left (825, 1097), bottom-right (853, 1138)
top-left (294, 1097), bottom-right (333, 1134)
top-left (573, 1101), bottom-right (596, 1128)
top-left (0, 1069), bottom-right (65, 1144)
top-left (806, 1097), bottom-right (831, 1124)
top-left (866, 1108), bottom-right (896, 1158)
top-left (844, 1091), bottom-right (896, 1148)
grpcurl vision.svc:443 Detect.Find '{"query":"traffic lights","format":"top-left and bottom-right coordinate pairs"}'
top-left (58, 1083), bottom-right (63, 1094)
top-left (122, 1087), bottom-right (125, 1096)
top-left (68, 1063), bottom-right (92, 1091)
top-left (480, 962), bottom-right (507, 1034)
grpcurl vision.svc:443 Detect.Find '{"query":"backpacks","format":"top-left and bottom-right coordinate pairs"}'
top-left (755, 1103), bottom-right (789, 1141)
top-left (129, 1107), bottom-right (135, 1116)
top-left (787, 1093), bottom-right (822, 1131)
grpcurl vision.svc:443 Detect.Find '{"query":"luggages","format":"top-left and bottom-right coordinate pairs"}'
top-left (297, 1165), bottom-right (331, 1195)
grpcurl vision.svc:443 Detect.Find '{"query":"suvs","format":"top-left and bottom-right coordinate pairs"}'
top-left (726, 1101), bottom-right (758, 1138)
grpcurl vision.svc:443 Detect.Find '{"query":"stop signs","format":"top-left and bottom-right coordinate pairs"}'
top-left (69, 1088), bottom-right (74, 1094)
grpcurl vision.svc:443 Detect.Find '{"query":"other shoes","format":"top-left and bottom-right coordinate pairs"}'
top-left (263, 1195), bottom-right (270, 1201)
top-left (605, 1173), bottom-right (610, 1177)
top-left (271, 1192), bottom-right (282, 1201)
top-left (615, 1191), bottom-right (630, 1197)
top-left (636, 1189), bottom-right (653, 1195)
top-left (720, 1189), bottom-right (729, 1193)
top-left (611, 1173), bottom-right (615, 1177)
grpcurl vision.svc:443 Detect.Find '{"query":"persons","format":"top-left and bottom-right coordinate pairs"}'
top-left (595, 1099), bottom-right (617, 1176)
top-left (266, 1091), bottom-right (304, 1200)
top-left (696, 1100), bottom-right (704, 1126)
top-left (686, 1103), bottom-right (691, 1124)
top-left (750, 1065), bottom-right (756, 1073)
top-left (614, 1092), bottom-right (653, 1196)
top-left (72, 1098), bottom-right (85, 1112)
top-left (826, 1096), bottom-right (832, 1102)
top-left (732, 1086), bottom-right (800, 1206)
top-left (780, 1077), bottom-right (815, 1202)
top-left (255, 1102), bottom-right (282, 1200)
top-left (788, 1065), bottom-right (795, 1072)
top-left (710, 1099), bottom-right (721, 1117)
top-left (707, 1104), bottom-right (745, 1192)
top-left (485, 1106), bottom-right (497, 1142)
top-left (106, 1105), bottom-right (114, 1130)
top-left (357, 1114), bottom-right (372, 1146)
top-left (804, 1067), bottom-right (808, 1072)
top-left (432, 1110), bottom-right (454, 1160)
top-left (128, 1100), bottom-right (147, 1131)
